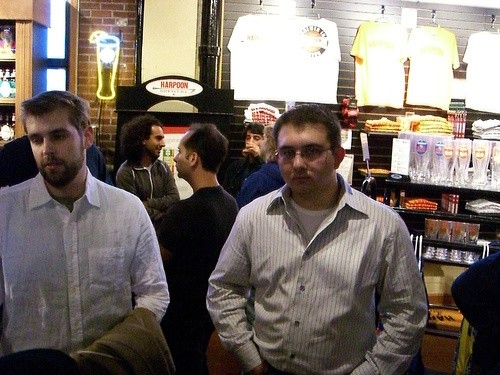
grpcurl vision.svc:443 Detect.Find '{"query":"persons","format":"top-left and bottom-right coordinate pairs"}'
top-left (1, 89), bottom-right (170, 375)
top-left (236, 126), bottom-right (289, 332)
top-left (116, 115), bottom-right (181, 227)
top-left (216, 123), bottom-right (268, 204)
top-left (153, 123), bottom-right (238, 375)
top-left (450, 251), bottom-right (500, 375)
top-left (205, 105), bottom-right (429, 375)
top-left (0, 97), bottom-right (115, 196)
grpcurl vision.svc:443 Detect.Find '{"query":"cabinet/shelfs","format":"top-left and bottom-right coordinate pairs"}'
top-left (0, 19), bottom-right (51, 149)
top-left (359, 115), bottom-right (500, 340)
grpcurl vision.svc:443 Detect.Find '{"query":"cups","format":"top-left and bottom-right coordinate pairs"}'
top-left (391, 130), bottom-right (500, 192)
top-left (246, 142), bottom-right (253, 152)
top-left (424, 218), bottom-right (480, 265)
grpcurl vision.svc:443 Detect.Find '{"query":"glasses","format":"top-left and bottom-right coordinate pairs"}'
top-left (274, 146), bottom-right (338, 158)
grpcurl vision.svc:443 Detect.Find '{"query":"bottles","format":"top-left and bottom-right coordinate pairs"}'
top-left (341, 95), bottom-right (360, 128)
top-left (446, 98), bottom-right (467, 138)
top-left (0, 111), bottom-right (15, 141)
top-left (0, 69), bottom-right (16, 98)
